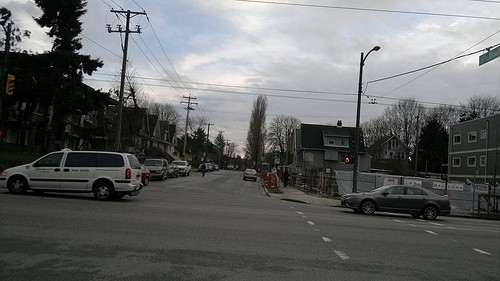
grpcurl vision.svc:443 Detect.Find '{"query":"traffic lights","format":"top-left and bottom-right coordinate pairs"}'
top-left (5, 73), bottom-right (15, 96)
top-left (344, 156), bottom-right (353, 164)
top-left (0, 129), bottom-right (6, 138)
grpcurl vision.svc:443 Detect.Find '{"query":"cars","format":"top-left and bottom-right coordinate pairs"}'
top-left (168, 164), bottom-right (179, 178)
top-left (139, 164), bottom-right (150, 186)
top-left (243, 169), bottom-right (257, 182)
top-left (341, 184), bottom-right (452, 221)
top-left (198, 163), bottom-right (220, 177)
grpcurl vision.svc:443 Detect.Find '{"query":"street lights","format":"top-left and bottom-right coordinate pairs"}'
top-left (115, 91), bottom-right (136, 151)
top-left (352, 45), bottom-right (381, 192)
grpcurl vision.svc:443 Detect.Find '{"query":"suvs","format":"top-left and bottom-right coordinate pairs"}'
top-left (0, 148), bottom-right (144, 202)
top-left (171, 160), bottom-right (192, 177)
top-left (143, 159), bottom-right (170, 181)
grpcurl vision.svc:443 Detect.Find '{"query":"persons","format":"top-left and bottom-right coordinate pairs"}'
top-left (200, 162), bottom-right (207, 176)
top-left (282, 170), bottom-right (291, 187)
